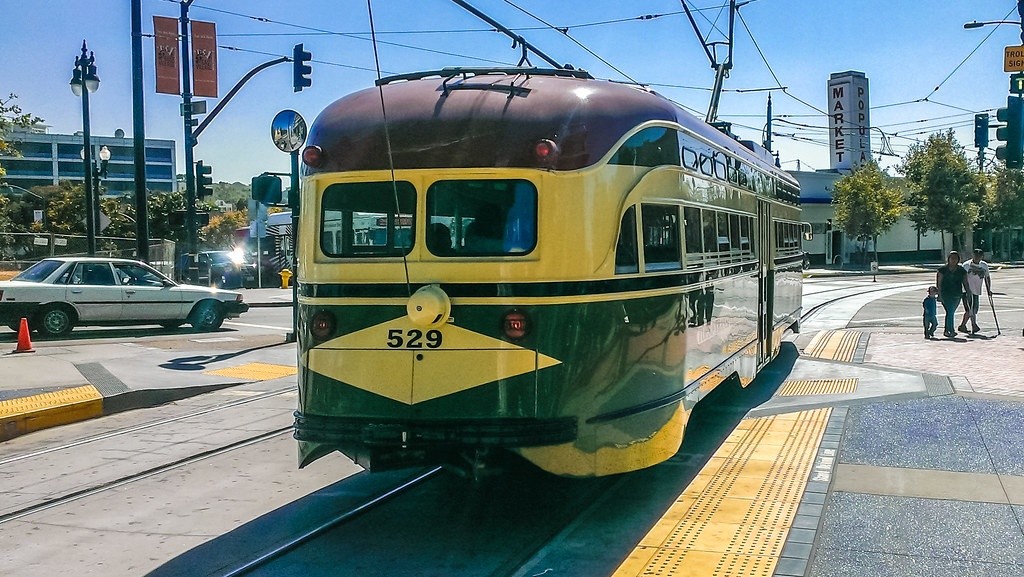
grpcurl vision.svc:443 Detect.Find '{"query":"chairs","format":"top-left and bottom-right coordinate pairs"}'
top-left (91, 265), bottom-right (107, 284)
top-left (427, 223), bottom-right (451, 256)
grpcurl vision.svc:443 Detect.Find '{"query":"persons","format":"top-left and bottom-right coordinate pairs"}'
top-left (923, 286), bottom-right (939, 339)
top-left (868, 237), bottom-right (873, 250)
top-left (937, 251), bottom-right (972, 337)
top-left (430, 223), bottom-right (453, 250)
top-left (685, 271), bottom-right (714, 326)
top-left (958, 249), bottom-right (992, 333)
top-left (463, 202), bottom-right (524, 252)
top-left (855, 236), bottom-right (862, 264)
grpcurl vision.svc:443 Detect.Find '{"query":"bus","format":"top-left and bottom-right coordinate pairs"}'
top-left (295, 0), bottom-right (813, 478)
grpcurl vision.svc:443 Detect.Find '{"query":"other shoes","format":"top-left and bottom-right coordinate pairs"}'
top-left (943, 331), bottom-right (950, 337)
top-left (972, 324), bottom-right (980, 332)
top-left (925, 335), bottom-right (930, 339)
top-left (951, 332), bottom-right (958, 337)
top-left (928, 331), bottom-right (934, 337)
top-left (957, 326), bottom-right (971, 333)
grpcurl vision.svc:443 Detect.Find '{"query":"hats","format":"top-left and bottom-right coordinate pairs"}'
top-left (947, 251), bottom-right (960, 264)
top-left (974, 249), bottom-right (983, 257)
top-left (927, 286), bottom-right (938, 292)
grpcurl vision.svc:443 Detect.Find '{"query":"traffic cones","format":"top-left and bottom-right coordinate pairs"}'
top-left (12, 318), bottom-right (36, 353)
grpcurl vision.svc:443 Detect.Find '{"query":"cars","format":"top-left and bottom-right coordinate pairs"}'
top-left (0, 257), bottom-right (249, 339)
top-left (175, 251), bottom-right (259, 289)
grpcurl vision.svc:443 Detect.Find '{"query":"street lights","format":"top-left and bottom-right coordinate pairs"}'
top-left (70, 39), bottom-right (100, 257)
top-left (81, 146), bottom-right (110, 252)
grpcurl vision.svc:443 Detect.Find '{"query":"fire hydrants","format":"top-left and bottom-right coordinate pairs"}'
top-left (279, 269), bottom-right (293, 288)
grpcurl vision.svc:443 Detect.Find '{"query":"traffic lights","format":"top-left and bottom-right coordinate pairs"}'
top-left (293, 40), bottom-right (312, 94)
top-left (252, 177), bottom-right (280, 205)
top-left (196, 160), bottom-right (213, 199)
top-left (974, 113), bottom-right (989, 148)
top-left (996, 96), bottom-right (1024, 168)
